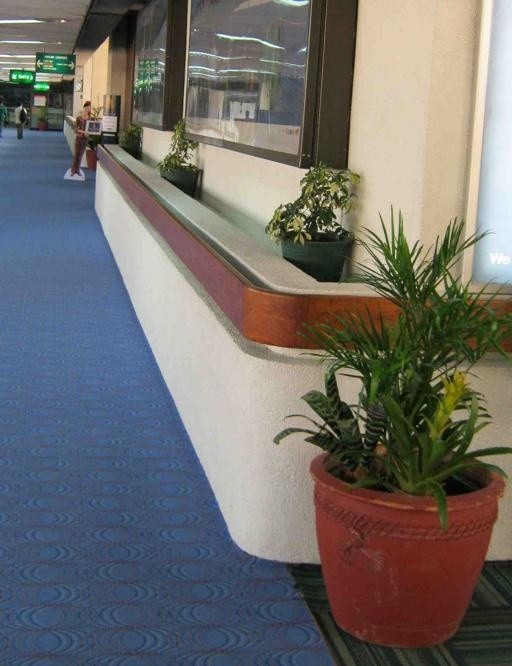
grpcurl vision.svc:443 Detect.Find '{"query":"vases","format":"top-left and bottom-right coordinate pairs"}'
top-left (281, 234), bottom-right (355, 282)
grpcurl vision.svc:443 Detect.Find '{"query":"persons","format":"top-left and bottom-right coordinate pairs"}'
top-left (71, 101), bottom-right (91, 176)
top-left (89, 123), bottom-right (99, 132)
top-left (15, 102), bottom-right (28, 139)
top-left (0, 99), bottom-right (7, 138)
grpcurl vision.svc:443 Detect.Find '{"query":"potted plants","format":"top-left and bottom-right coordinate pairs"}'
top-left (117, 123), bottom-right (143, 158)
top-left (153, 117), bottom-right (201, 198)
top-left (36, 107), bottom-right (49, 131)
top-left (273, 203), bottom-right (511, 650)
top-left (86, 136), bottom-right (97, 170)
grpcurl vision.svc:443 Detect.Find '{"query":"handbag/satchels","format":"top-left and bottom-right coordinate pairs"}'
top-left (20, 109), bottom-right (26, 122)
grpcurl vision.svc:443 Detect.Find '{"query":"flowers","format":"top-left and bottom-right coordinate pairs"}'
top-left (265, 159), bottom-right (360, 247)
